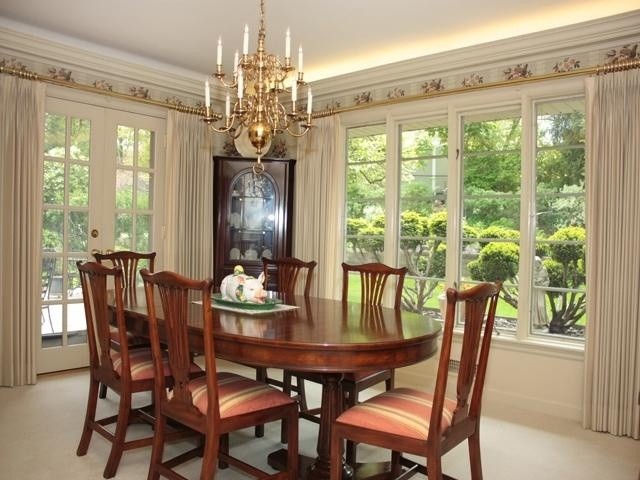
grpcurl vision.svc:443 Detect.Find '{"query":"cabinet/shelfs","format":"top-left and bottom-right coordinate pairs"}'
top-left (212, 155), bottom-right (296, 293)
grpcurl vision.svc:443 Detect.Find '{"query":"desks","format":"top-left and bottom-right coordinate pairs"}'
top-left (105, 286), bottom-right (442, 480)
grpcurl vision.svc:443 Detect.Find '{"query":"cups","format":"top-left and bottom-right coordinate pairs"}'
top-left (264, 291), bottom-right (286, 305)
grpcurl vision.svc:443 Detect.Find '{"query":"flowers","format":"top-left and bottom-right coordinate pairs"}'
top-left (438, 284), bottom-right (470, 297)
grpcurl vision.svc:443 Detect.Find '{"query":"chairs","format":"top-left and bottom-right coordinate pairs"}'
top-left (282, 262), bottom-right (407, 466)
top-left (93, 250), bottom-right (156, 399)
top-left (140, 267), bottom-right (299, 480)
top-left (255, 255), bottom-right (317, 439)
top-left (41, 245), bottom-right (54, 334)
top-left (330, 280), bottom-right (502, 479)
top-left (76, 259), bottom-right (206, 480)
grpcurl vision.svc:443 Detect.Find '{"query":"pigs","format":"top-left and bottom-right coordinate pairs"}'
top-left (220, 271), bottom-right (267, 305)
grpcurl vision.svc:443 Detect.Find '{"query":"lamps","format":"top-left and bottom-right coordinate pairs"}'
top-left (201, 0), bottom-right (312, 166)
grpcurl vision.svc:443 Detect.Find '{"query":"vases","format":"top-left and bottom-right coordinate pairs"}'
top-left (438, 293), bottom-right (465, 323)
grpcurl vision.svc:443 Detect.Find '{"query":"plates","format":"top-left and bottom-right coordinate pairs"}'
top-left (211, 294), bottom-right (283, 309)
